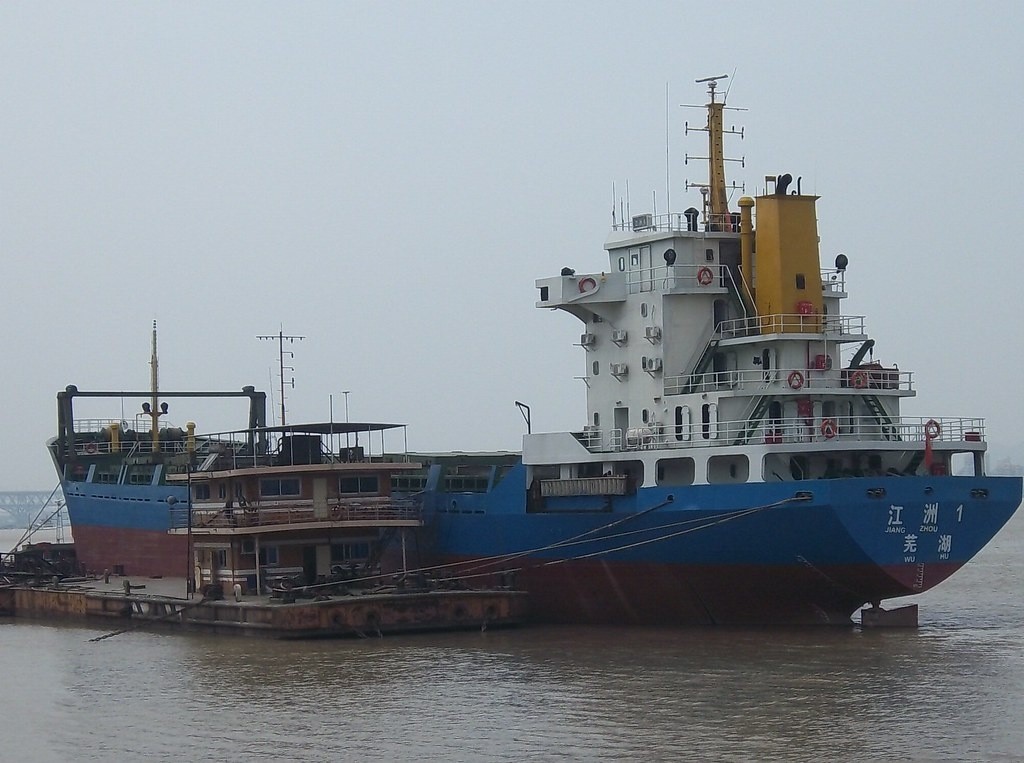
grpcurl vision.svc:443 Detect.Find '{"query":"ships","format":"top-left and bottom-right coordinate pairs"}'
top-left (0, 67), bottom-right (1023, 639)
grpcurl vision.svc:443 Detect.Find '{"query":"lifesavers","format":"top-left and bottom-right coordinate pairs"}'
top-left (329, 505), bottom-right (343, 520)
top-left (788, 372), bottom-right (805, 390)
top-left (924, 420), bottom-right (940, 438)
top-left (579, 277), bottom-right (596, 292)
top-left (698, 267), bottom-right (714, 285)
top-left (852, 371), bottom-right (867, 387)
top-left (85, 443), bottom-right (97, 453)
top-left (821, 419), bottom-right (836, 438)
